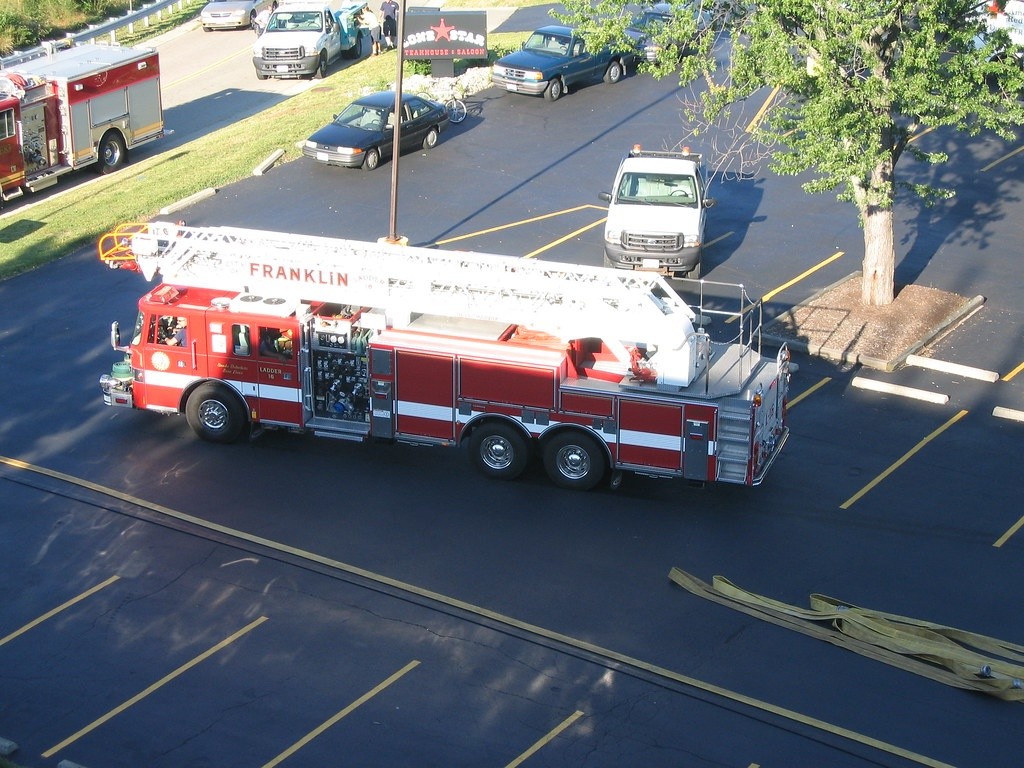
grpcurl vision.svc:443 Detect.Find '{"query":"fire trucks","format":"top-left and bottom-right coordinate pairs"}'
top-left (98, 219), bottom-right (799, 494)
top-left (0, 41), bottom-right (175, 212)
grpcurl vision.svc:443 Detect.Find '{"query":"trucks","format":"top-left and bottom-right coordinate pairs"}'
top-left (252, 0), bottom-right (370, 80)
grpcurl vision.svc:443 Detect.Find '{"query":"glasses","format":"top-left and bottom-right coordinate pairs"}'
top-left (177, 320), bottom-right (185, 322)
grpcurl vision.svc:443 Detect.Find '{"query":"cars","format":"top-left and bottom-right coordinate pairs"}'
top-left (200, 0), bottom-right (280, 32)
top-left (301, 90), bottom-right (450, 172)
top-left (618, 3), bottom-right (712, 66)
top-left (492, 25), bottom-right (638, 103)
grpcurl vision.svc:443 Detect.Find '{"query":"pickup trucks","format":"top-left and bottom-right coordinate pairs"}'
top-left (599, 143), bottom-right (716, 281)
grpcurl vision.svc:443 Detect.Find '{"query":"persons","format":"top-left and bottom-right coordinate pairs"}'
top-left (254, 4), bottom-right (277, 36)
top-left (378, 0), bottom-right (399, 49)
top-left (358, 7), bottom-right (382, 55)
top-left (164, 317), bottom-right (188, 347)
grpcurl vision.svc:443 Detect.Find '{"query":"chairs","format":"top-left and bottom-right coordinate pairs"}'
top-left (360, 110), bottom-right (402, 130)
top-left (314, 17), bottom-right (322, 27)
top-left (636, 180), bottom-right (694, 198)
top-left (239, 325), bottom-right (250, 347)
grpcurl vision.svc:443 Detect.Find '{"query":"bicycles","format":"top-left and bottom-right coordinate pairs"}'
top-left (445, 78), bottom-right (468, 124)
